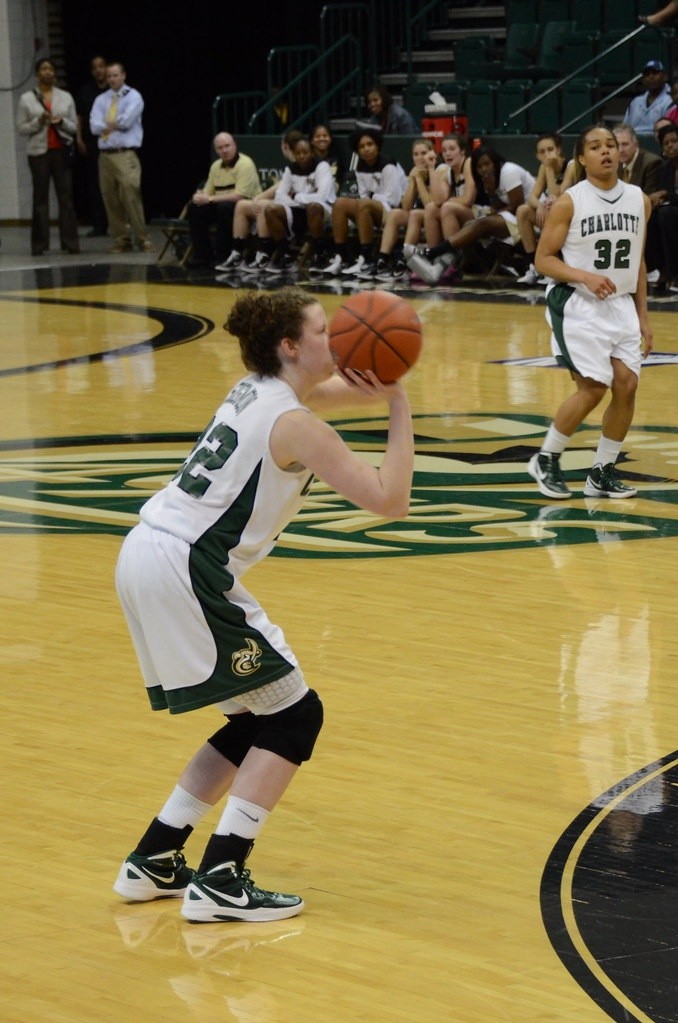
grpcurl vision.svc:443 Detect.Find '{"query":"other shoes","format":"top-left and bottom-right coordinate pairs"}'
top-left (86, 227), bottom-right (107, 238)
top-left (647, 269), bottom-right (678, 292)
top-left (60, 243), bottom-right (79, 254)
top-left (638, 14), bottom-right (655, 29)
top-left (110, 243), bottom-right (133, 252)
top-left (179, 257), bottom-right (200, 275)
top-left (32, 243), bottom-right (48, 255)
top-left (138, 240), bottom-right (157, 252)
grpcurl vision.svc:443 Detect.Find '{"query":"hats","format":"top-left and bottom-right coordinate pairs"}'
top-left (641, 59), bottom-right (665, 71)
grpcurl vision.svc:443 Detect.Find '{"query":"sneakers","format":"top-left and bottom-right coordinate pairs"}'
top-left (181, 862), bottom-right (305, 922)
top-left (530, 503), bottom-right (572, 540)
top-left (583, 463), bottom-right (637, 499)
top-left (527, 452), bottom-right (573, 498)
top-left (214, 242), bottom-right (463, 285)
top-left (585, 497), bottom-right (637, 536)
top-left (215, 273), bottom-right (452, 292)
top-left (517, 264), bottom-right (558, 285)
top-left (112, 847), bottom-right (197, 901)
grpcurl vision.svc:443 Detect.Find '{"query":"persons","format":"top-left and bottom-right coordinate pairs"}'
top-left (622, 60), bottom-right (674, 133)
top-left (17, 57), bottom-right (84, 258)
top-left (529, 501), bottom-right (665, 866)
top-left (187, 116), bottom-right (678, 299)
top-left (113, 293), bottom-right (416, 924)
top-left (359, 87), bottom-right (419, 137)
top-left (75, 57), bottom-right (112, 238)
top-left (526, 127), bottom-right (653, 499)
top-left (665, 79), bottom-right (678, 123)
top-left (89, 64), bottom-right (154, 254)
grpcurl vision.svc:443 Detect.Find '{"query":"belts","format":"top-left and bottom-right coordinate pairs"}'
top-left (101, 148), bottom-right (135, 153)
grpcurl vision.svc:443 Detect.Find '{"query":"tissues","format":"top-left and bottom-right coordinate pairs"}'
top-left (424, 91), bottom-right (457, 112)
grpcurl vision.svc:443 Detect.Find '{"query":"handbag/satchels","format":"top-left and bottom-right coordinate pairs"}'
top-left (64, 134), bottom-right (79, 161)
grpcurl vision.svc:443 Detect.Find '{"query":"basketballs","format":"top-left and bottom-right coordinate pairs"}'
top-left (329, 290), bottom-right (422, 383)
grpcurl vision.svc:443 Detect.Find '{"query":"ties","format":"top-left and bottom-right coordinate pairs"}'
top-left (101, 94), bottom-right (118, 141)
top-left (623, 167), bottom-right (629, 183)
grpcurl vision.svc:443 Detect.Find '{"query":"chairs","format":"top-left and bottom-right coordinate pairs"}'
top-left (403, 1), bottom-right (678, 135)
top-left (149, 201), bottom-right (195, 268)
